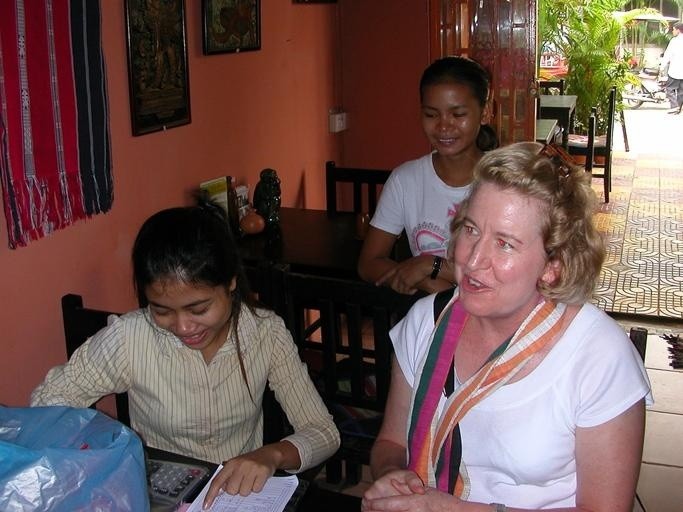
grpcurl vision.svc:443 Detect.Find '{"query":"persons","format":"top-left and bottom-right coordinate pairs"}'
top-left (659, 24), bottom-right (683, 114)
top-left (29, 206), bottom-right (342, 510)
top-left (358, 57), bottom-right (490, 295)
top-left (362, 142), bottom-right (654, 512)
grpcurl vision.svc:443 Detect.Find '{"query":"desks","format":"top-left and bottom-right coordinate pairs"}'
top-left (143, 443), bottom-right (362, 512)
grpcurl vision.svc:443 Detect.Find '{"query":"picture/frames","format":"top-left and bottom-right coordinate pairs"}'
top-left (123, 1), bottom-right (192, 138)
top-left (202, 0), bottom-right (262, 57)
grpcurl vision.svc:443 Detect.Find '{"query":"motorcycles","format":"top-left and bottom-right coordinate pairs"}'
top-left (622, 68), bottom-right (678, 109)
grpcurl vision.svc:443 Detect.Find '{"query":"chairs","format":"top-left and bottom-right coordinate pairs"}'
top-left (537, 79), bottom-right (616, 205)
top-left (60, 161), bottom-right (407, 447)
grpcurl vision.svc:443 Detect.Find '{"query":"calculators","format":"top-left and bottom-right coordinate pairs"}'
top-left (145, 457), bottom-right (210, 512)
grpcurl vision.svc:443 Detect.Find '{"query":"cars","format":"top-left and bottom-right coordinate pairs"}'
top-left (539, 52), bottom-right (568, 81)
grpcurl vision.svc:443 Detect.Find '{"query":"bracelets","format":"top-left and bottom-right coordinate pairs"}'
top-left (431, 256), bottom-right (442, 279)
top-left (495, 504), bottom-right (505, 512)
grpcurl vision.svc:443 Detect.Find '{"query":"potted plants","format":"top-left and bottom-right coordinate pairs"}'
top-left (551, 9), bottom-right (640, 165)
